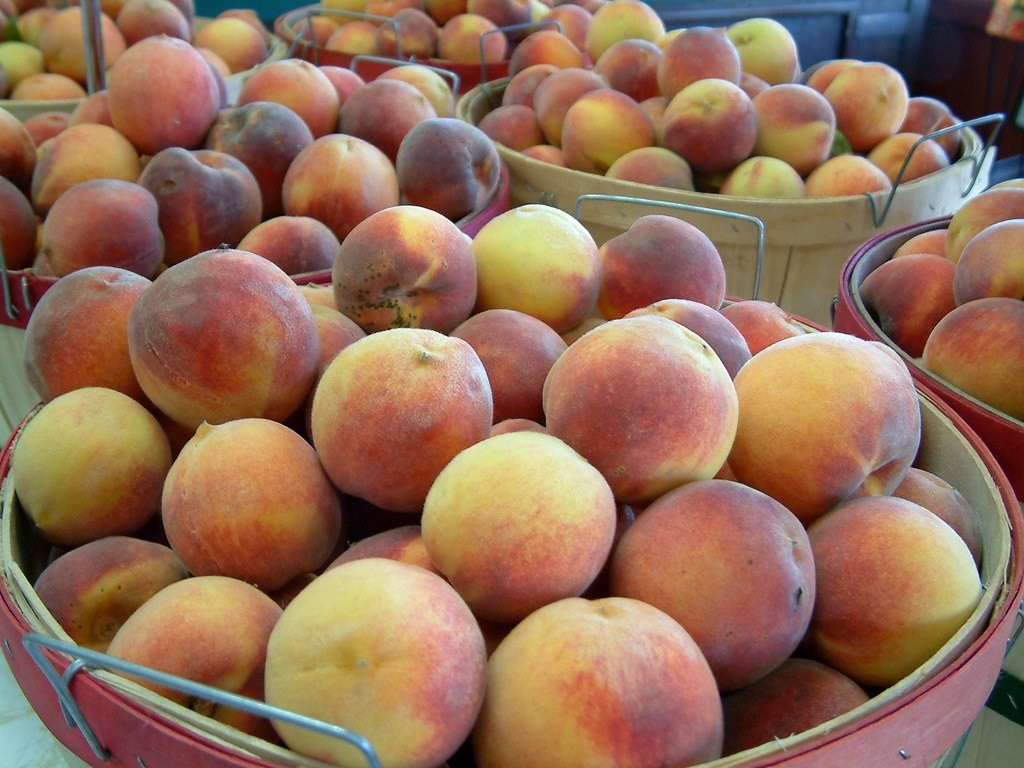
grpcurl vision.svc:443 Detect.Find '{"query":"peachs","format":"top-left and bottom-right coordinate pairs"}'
top-left (0, 0), bottom-right (1024, 768)
